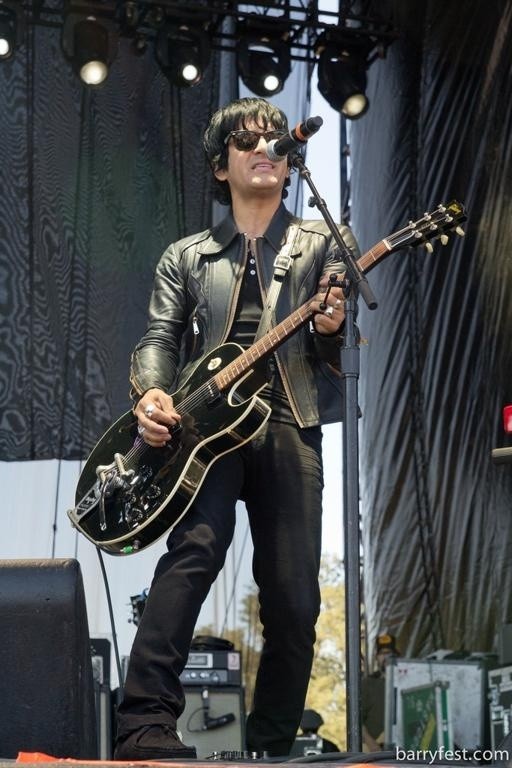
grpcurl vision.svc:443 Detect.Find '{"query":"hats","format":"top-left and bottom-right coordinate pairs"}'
top-left (300, 709), bottom-right (321, 731)
top-left (377, 635), bottom-right (397, 657)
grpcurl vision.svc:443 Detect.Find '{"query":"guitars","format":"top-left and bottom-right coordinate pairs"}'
top-left (69, 201), bottom-right (466, 555)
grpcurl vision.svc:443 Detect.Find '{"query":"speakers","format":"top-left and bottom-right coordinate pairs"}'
top-left (94, 689), bottom-right (112, 760)
top-left (175, 686), bottom-right (246, 761)
top-left (0, 557), bottom-right (101, 762)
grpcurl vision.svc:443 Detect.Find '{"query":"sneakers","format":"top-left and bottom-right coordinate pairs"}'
top-left (113, 726), bottom-right (197, 760)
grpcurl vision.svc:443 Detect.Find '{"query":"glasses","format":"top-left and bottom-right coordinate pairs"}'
top-left (224, 129), bottom-right (286, 152)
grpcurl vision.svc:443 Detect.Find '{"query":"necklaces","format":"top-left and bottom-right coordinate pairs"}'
top-left (243, 232), bottom-right (257, 243)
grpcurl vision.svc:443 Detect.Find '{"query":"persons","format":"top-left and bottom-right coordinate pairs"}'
top-left (112, 94), bottom-right (362, 762)
top-left (360, 633), bottom-right (405, 752)
top-left (296, 708), bottom-right (341, 753)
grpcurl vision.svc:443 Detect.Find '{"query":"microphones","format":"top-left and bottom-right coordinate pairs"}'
top-left (265, 116), bottom-right (324, 162)
top-left (202, 713), bottom-right (235, 731)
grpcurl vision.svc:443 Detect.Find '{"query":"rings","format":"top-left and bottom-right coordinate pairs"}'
top-left (144, 403), bottom-right (156, 419)
top-left (324, 305), bottom-right (333, 317)
top-left (136, 424), bottom-right (145, 435)
top-left (335, 298), bottom-right (342, 309)
